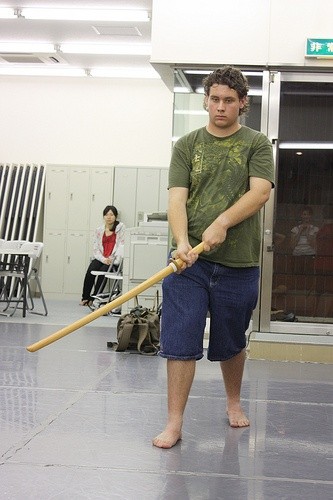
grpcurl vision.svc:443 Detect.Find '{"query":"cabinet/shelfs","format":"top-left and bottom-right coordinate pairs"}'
top-left (38, 162), bottom-right (170, 302)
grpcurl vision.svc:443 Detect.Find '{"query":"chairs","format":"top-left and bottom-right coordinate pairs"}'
top-left (86, 243), bottom-right (124, 317)
top-left (0, 238), bottom-right (48, 318)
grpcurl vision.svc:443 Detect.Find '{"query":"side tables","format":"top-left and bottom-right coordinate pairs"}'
top-left (120, 226), bottom-right (169, 317)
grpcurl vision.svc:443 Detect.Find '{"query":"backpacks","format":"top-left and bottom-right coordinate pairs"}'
top-left (108, 304), bottom-right (161, 356)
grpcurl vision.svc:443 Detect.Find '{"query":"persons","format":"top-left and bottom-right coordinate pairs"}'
top-left (79, 206), bottom-right (126, 306)
top-left (289, 207), bottom-right (318, 291)
top-left (152, 67), bottom-right (275, 449)
top-left (314, 213), bottom-right (333, 291)
